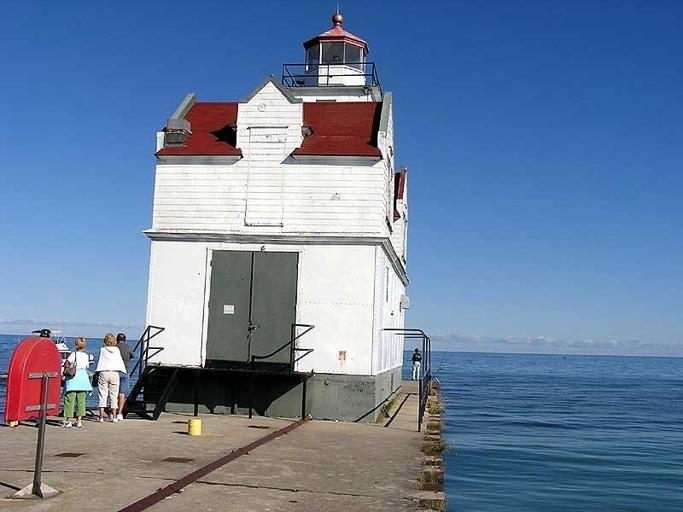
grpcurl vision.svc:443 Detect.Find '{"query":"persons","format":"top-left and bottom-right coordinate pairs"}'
top-left (94, 332), bottom-right (127, 424)
top-left (59, 335), bottom-right (94, 430)
top-left (408, 347), bottom-right (422, 381)
top-left (114, 332), bottom-right (135, 422)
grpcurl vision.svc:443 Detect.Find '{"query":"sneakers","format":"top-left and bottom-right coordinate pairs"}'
top-left (98, 413), bottom-right (124, 424)
top-left (60, 420), bottom-right (83, 428)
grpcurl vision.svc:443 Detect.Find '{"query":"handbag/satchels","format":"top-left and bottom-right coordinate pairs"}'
top-left (91, 373), bottom-right (98, 386)
top-left (61, 351), bottom-right (78, 379)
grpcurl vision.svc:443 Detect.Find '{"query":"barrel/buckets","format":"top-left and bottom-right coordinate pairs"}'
top-left (188, 418), bottom-right (202, 436)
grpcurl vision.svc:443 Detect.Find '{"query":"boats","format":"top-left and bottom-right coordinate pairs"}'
top-left (54, 337), bottom-right (71, 378)
